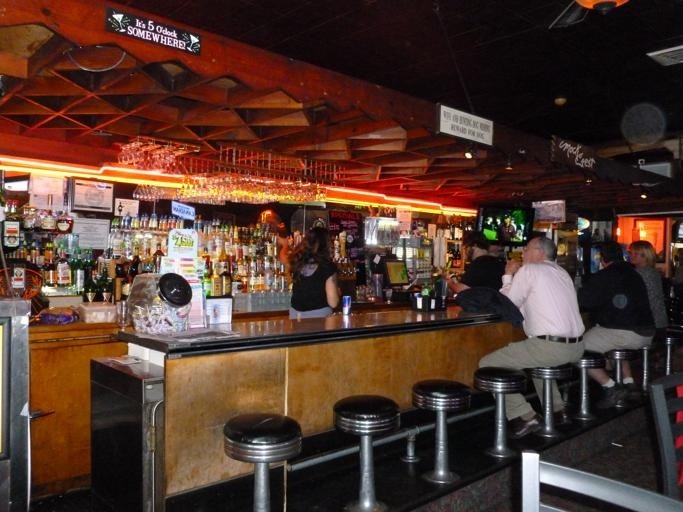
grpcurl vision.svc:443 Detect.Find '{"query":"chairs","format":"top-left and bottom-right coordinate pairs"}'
top-left (522, 450), bottom-right (681, 511)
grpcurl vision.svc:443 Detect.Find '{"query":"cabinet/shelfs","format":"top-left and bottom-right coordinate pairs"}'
top-left (445, 239), bottom-right (465, 274)
top-left (392, 238), bottom-right (434, 286)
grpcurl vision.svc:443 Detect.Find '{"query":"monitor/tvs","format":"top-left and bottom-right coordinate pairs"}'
top-left (383, 259), bottom-right (410, 285)
top-left (476, 204), bottom-right (535, 247)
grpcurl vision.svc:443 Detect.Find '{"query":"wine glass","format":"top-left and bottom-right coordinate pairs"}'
top-left (114, 142), bottom-right (176, 172)
top-left (129, 173), bottom-right (327, 209)
top-left (383, 288), bottom-right (392, 304)
top-left (115, 301), bottom-right (130, 332)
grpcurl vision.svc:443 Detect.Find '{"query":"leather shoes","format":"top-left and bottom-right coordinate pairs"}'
top-left (595, 386), bottom-right (626, 408)
top-left (511, 413), bottom-right (544, 440)
top-left (555, 409), bottom-right (572, 428)
top-left (626, 383), bottom-right (640, 402)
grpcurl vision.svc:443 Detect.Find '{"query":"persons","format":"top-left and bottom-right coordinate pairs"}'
top-left (605, 242), bottom-right (669, 372)
top-left (577, 241), bottom-right (656, 409)
top-left (498, 211), bottom-right (516, 243)
top-left (277, 227), bottom-right (339, 319)
top-left (479, 236), bottom-right (584, 439)
top-left (442, 231), bottom-right (505, 304)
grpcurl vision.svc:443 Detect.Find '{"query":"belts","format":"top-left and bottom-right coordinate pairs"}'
top-left (537, 336), bottom-right (583, 343)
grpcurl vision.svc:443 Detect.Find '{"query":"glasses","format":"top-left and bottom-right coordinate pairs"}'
top-left (523, 246), bottom-right (538, 251)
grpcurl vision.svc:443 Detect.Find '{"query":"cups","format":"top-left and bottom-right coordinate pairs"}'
top-left (414, 294), bottom-right (447, 311)
top-left (371, 274), bottom-right (383, 298)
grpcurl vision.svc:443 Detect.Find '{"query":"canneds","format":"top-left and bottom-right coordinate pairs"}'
top-left (343, 315), bottom-right (351, 330)
top-left (341, 295), bottom-right (352, 315)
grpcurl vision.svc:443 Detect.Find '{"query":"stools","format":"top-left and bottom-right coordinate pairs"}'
top-left (332, 395), bottom-right (402, 512)
top-left (531, 366), bottom-right (574, 439)
top-left (412, 379), bottom-right (473, 486)
top-left (223, 413), bottom-right (303, 511)
top-left (578, 357), bottom-right (606, 420)
top-left (608, 349), bottom-right (635, 407)
top-left (475, 366), bottom-right (524, 460)
top-left (637, 343), bottom-right (660, 392)
top-left (658, 334), bottom-right (681, 380)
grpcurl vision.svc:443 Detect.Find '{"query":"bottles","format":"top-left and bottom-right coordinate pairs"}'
top-left (421, 280), bottom-right (431, 296)
top-left (190, 214), bottom-right (285, 294)
top-left (412, 221), bottom-right (423, 235)
top-left (435, 213), bottom-right (474, 240)
top-left (111, 210), bottom-right (183, 232)
top-left (352, 286), bottom-right (370, 301)
top-left (447, 243), bottom-right (461, 267)
top-left (573, 269), bottom-right (580, 285)
top-left (0, 192), bottom-right (73, 234)
top-left (332, 227), bottom-right (347, 275)
top-left (3, 234), bottom-right (161, 303)
